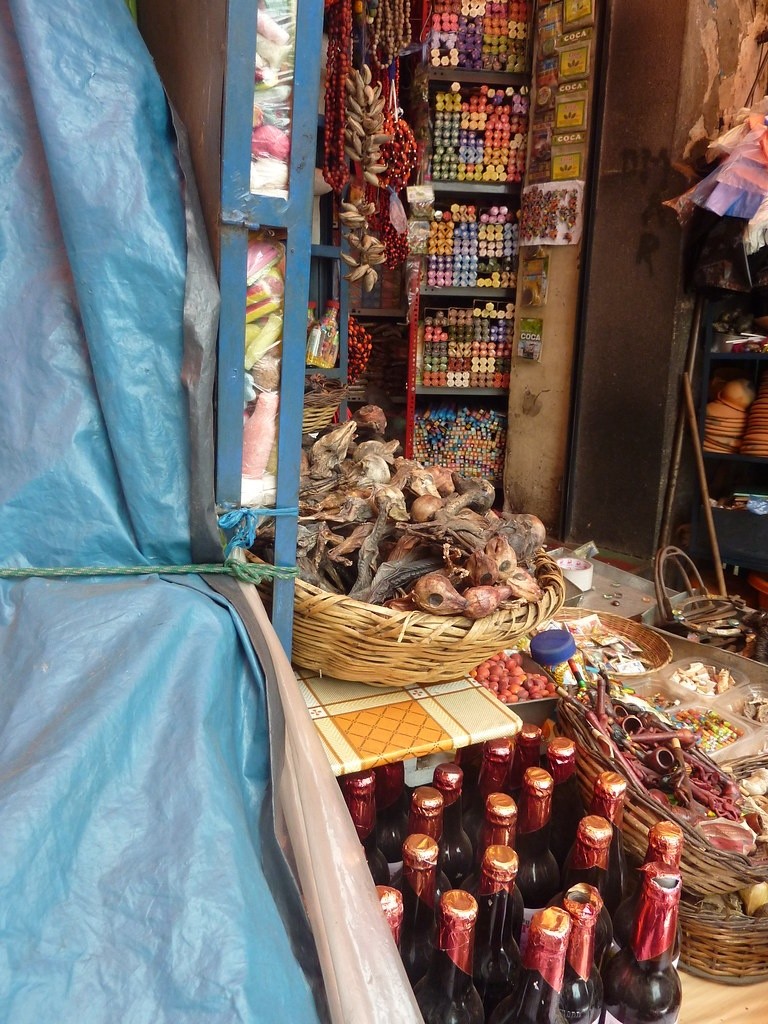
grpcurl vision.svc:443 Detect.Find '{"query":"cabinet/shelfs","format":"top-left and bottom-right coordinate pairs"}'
top-left (676, 264), bottom-right (768, 596)
top-left (226, 0), bottom-right (541, 668)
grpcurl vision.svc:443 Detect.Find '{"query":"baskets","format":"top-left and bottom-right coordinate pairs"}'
top-left (557, 683), bottom-right (768, 896)
top-left (678, 899), bottom-right (768, 985)
top-left (530, 607), bottom-right (673, 684)
top-left (246, 520), bottom-right (565, 685)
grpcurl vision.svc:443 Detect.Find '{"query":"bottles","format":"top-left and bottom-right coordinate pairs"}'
top-left (344, 718), bottom-right (694, 1024)
top-left (530, 630), bottom-right (589, 686)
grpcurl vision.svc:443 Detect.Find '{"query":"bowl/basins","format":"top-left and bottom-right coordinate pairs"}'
top-left (703, 373), bottom-right (768, 457)
